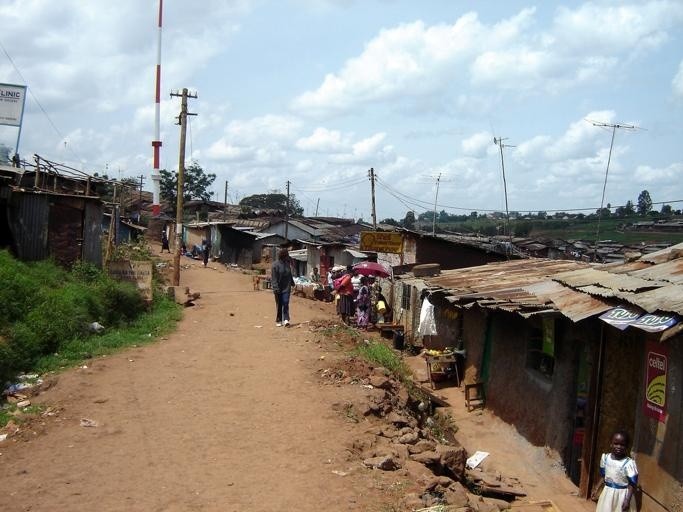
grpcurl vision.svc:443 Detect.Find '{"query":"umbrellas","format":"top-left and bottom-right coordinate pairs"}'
top-left (353, 261), bottom-right (390, 281)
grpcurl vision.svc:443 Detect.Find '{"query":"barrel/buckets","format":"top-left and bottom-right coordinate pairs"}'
top-left (377, 300), bottom-right (386, 313)
top-left (393, 325), bottom-right (404, 349)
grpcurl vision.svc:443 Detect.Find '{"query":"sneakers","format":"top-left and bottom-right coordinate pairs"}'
top-left (276, 320), bottom-right (289, 327)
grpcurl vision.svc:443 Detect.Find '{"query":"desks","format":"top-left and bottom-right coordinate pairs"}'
top-left (252, 275), bottom-right (272, 291)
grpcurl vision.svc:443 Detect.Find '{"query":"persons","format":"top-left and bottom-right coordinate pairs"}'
top-left (203, 246), bottom-right (208, 266)
top-left (191, 245), bottom-right (197, 256)
top-left (330, 268), bottom-right (357, 321)
top-left (589, 429), bottom-right (639, 512)
top-left (310, 267), bottom-right (320, 282)
top-left (372, 285), bottom-right (391, 322)
top-left (269, 248), bottom-right (294, 328)
top-left (158, 234), bottom-right (169, 253)
top-left (352, 275), bottom-right (373, 328)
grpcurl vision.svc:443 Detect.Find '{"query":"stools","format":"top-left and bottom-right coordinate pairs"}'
top-left (464, 377), bottom-right (486, 413)
